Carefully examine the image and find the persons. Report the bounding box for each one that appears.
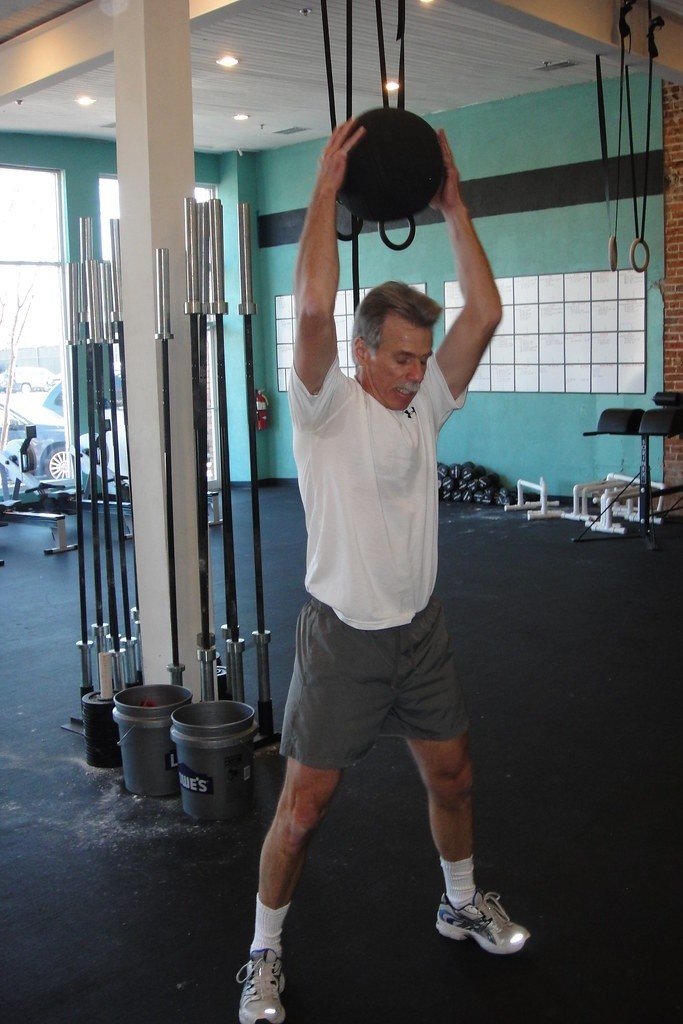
[233,118,533,1024]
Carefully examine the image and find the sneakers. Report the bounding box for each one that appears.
[435,887,532,956]
[235,947,286,1024]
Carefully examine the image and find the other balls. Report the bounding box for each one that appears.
[338,107,444,221]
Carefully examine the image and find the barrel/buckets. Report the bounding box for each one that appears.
[170,700,260,822]
[111,683,193,797]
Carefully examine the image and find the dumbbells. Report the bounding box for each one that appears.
[437,461,539,506]
